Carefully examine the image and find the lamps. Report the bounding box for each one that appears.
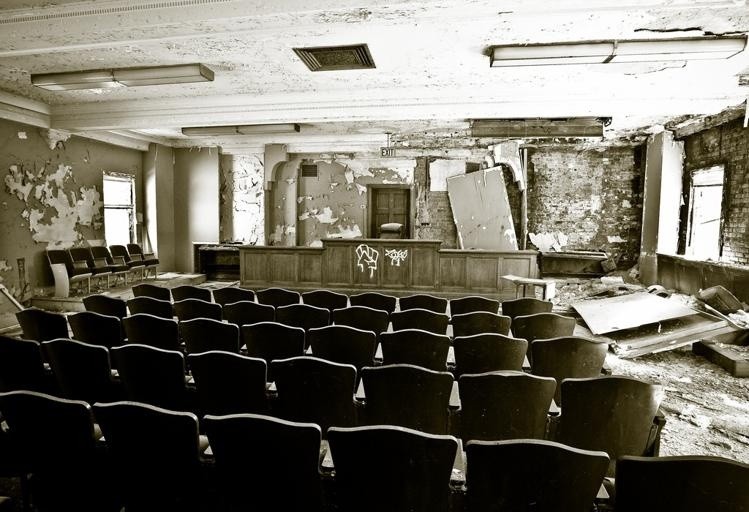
[31,63,214,91]
[490,35,748,67]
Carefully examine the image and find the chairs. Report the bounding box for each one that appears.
[2,387,748,510]
[47,243,162,298]
[3,282,667,460]
